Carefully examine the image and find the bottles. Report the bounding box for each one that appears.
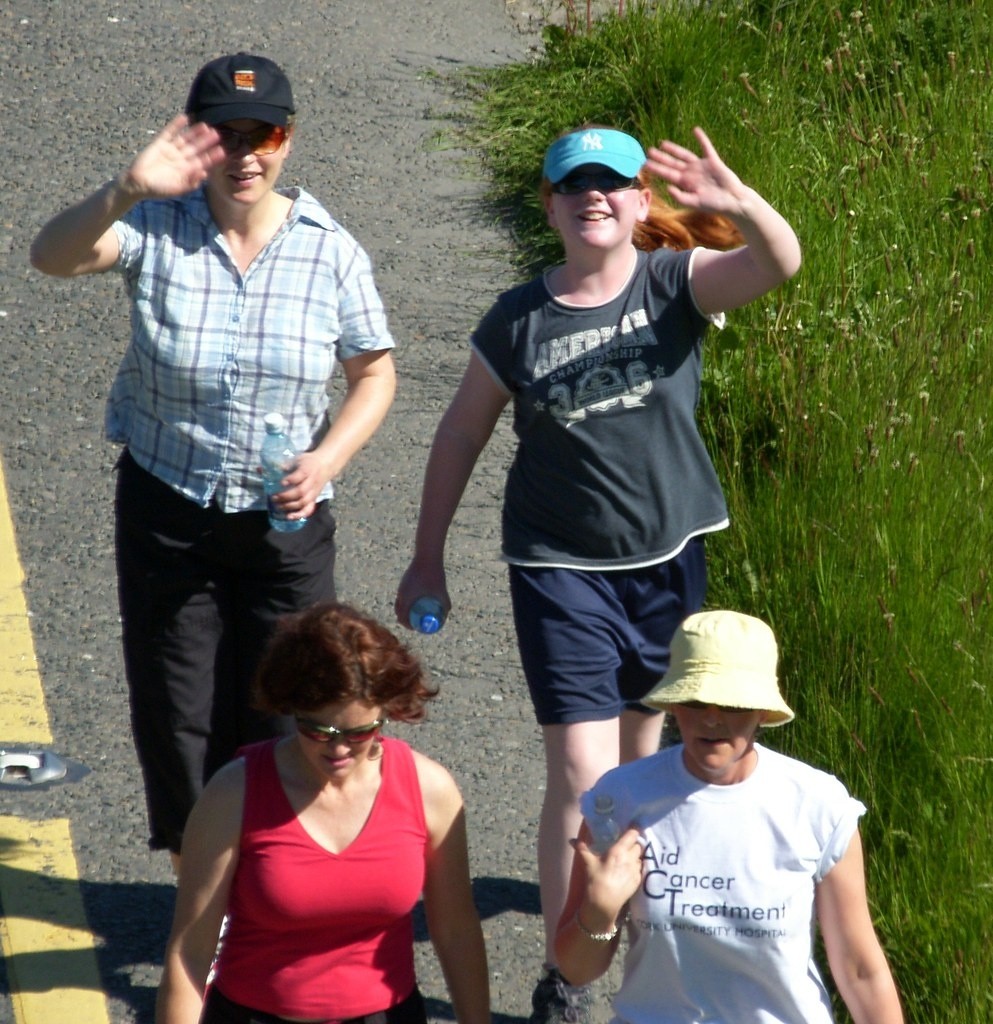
[409,597,445,634]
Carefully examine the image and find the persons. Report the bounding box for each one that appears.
[394,125,801,1024]
[32,52,397,985]
[153,604,491,1024]
[554,610,904,1024]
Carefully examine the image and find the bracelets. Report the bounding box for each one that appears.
[576,909,617,941]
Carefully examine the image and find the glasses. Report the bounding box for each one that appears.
[552,170,639,194]
[295,703,384,743]
[219,125,286,156]
[677,701,754,714]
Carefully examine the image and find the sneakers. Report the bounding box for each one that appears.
[529,963,592,1024]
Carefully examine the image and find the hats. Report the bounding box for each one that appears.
[542,129,646,183]
[639,610,795,727]
[185,50,296,127]
